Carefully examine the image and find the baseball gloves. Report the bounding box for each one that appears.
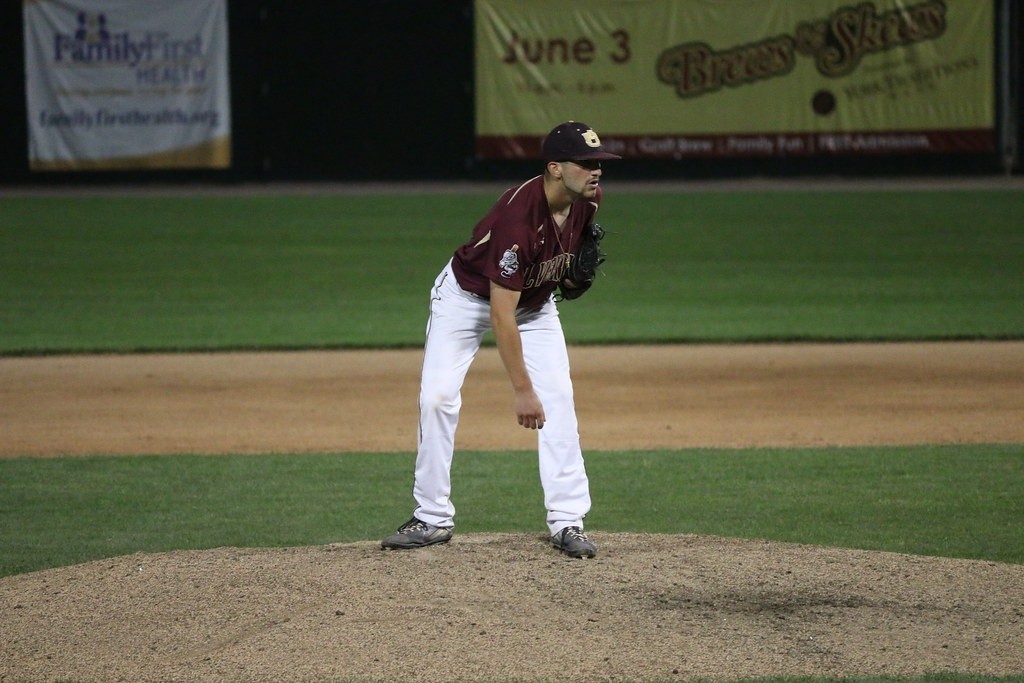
[551,221,608,303]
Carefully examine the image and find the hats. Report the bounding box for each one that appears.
[540,120,623,162]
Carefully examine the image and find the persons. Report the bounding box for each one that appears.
[380,120,621,558]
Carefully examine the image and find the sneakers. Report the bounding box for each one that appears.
[380,516,453,549]
[548,526,598,560]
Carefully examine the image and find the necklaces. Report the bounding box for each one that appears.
[544,178,575,268]
